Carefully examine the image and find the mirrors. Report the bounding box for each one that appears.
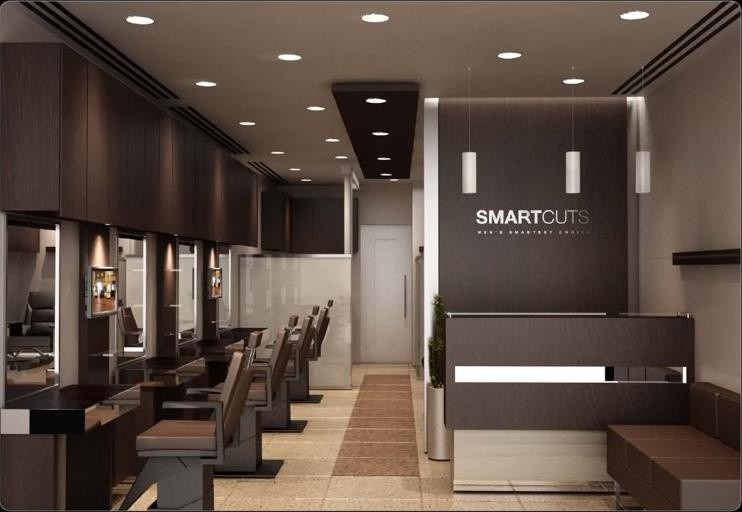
[216,244,232,330]
[112,232,149,356]
[0,214,61,405]
[175,238,198,345]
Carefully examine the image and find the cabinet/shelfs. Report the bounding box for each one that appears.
[0,41,345,254]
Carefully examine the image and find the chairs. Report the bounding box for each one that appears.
[135,300,334,511]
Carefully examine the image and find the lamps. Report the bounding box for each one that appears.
[461,67,477,194]
[565,66,581,194]
[636,64,652,194]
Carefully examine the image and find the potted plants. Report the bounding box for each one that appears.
[427,295,455,462]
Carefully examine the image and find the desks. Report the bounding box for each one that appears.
[0,382,142,512]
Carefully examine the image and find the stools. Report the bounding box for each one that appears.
[606,422,742,512]
